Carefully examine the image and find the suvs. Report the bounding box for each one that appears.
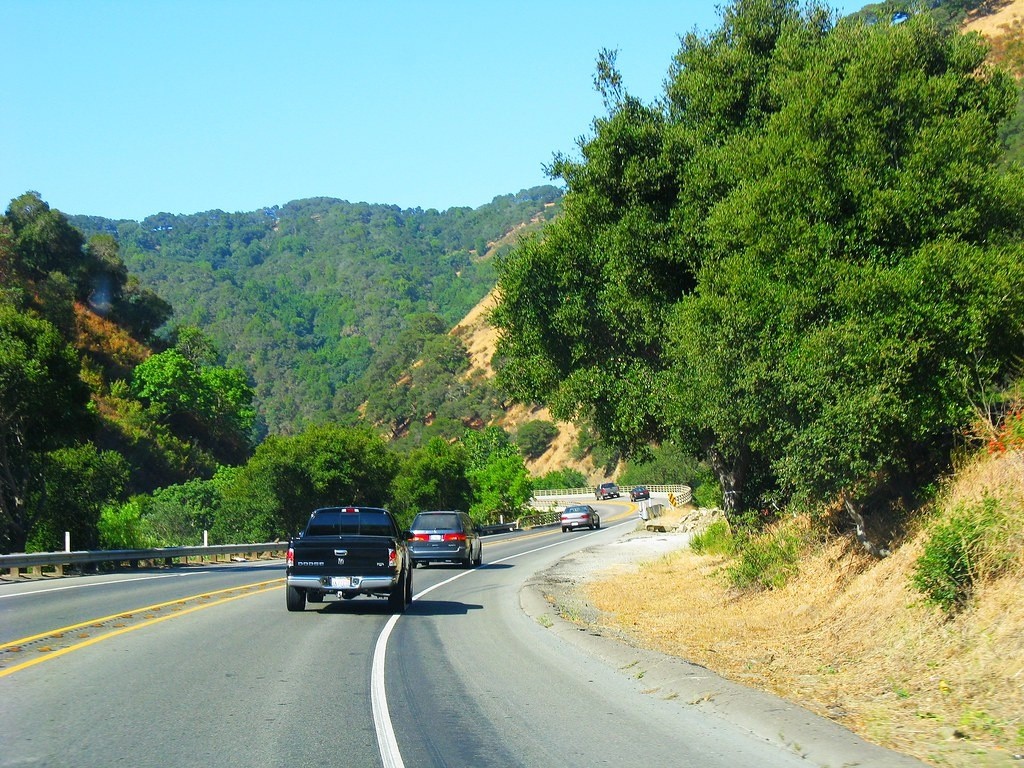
[407,510,482,568]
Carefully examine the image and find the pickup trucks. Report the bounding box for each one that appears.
[286,507,413,612]
[595,482,620,500]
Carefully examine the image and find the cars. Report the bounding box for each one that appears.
[630,487,650,502]
[560,505,601,532]
